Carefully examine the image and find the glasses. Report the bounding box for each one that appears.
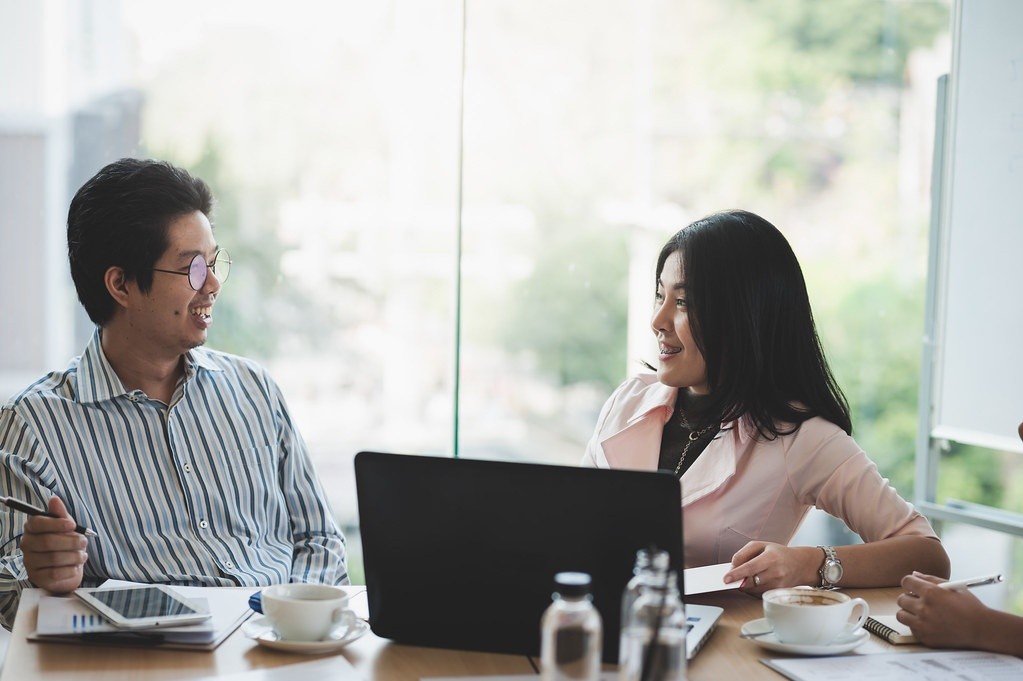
[145,248,232,291]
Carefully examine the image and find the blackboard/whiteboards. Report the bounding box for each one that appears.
[926,0,1023,457]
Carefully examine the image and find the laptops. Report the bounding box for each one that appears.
[353,450,723,661]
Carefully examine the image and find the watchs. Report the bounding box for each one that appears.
[817,545,844,590]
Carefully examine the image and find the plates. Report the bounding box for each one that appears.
[244,616,369,655]
[743,618,869,656]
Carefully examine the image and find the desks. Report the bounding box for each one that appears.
[0,584,1023,681]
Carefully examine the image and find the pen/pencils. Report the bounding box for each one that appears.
[0,494,99,538]
[936,574,1004,587]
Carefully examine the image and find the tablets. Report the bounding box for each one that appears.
[73,583,213,629]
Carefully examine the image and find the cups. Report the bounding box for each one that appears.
[260,583,357,643]
[761,587,869,644]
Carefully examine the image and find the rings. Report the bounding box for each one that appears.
[754,575,760,586]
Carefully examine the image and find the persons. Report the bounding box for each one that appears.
[896,571,1023,657]
[581,212,953,597]
[0,159,350,634]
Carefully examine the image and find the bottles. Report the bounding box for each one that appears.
[540,572,603,681]
[618,549,686,681]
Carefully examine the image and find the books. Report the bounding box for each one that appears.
[857,615,922,645]
[24,579,265,652]
